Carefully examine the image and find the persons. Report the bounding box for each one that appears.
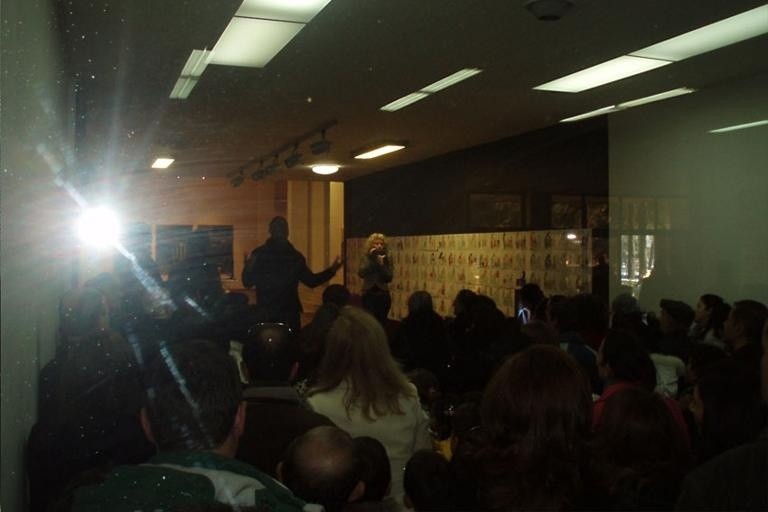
[26,215,768,512]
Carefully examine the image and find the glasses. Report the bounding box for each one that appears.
[227,119,339,187]
